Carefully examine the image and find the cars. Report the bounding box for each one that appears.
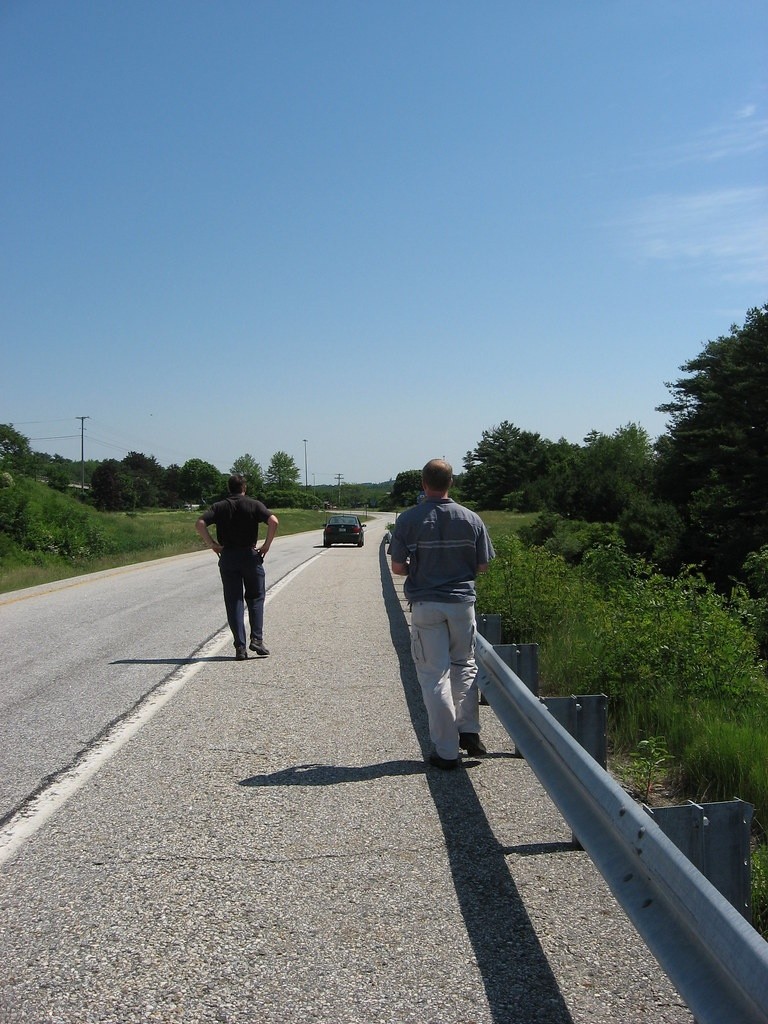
[322,515,367,547]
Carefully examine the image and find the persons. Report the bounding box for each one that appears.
[195,474,279,660]
[386,459,496,768]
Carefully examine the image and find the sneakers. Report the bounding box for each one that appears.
[249,639,270,655]
[235,647,247,660]
[458,732,487,756]
[429,752,458,770]
